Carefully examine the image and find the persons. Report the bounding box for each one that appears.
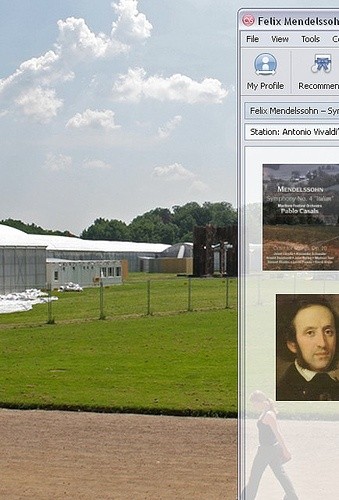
[277,300,339,401]
[237,391,299,500]
